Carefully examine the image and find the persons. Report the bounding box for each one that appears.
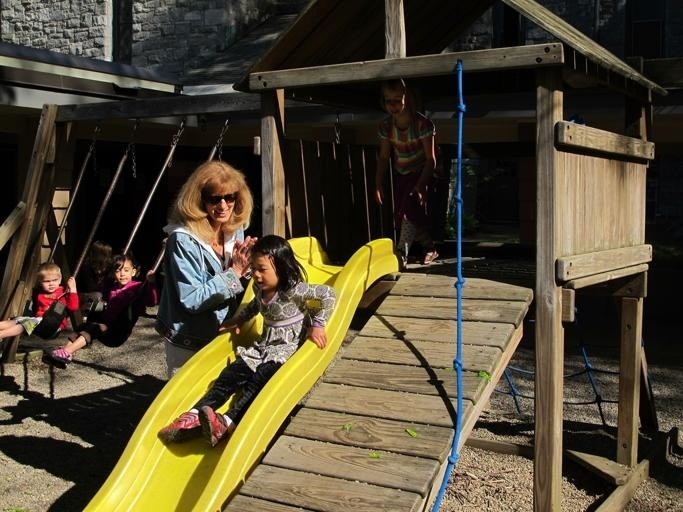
[372,79,439,266]
[48,254,160,365]
[0,262,80,341]
[158,232,337,454]
[151,155,258,383]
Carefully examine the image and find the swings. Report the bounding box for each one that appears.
[23,118,139,347]
[69,117,234,348]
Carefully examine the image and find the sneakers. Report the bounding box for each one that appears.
[401,256,409,265]
[423,252,439,264]
[43,346,72,369]
[158,405,228,448]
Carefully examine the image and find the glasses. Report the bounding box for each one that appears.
[207,194,235,205]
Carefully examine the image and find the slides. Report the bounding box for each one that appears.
[84,235,400,512]
